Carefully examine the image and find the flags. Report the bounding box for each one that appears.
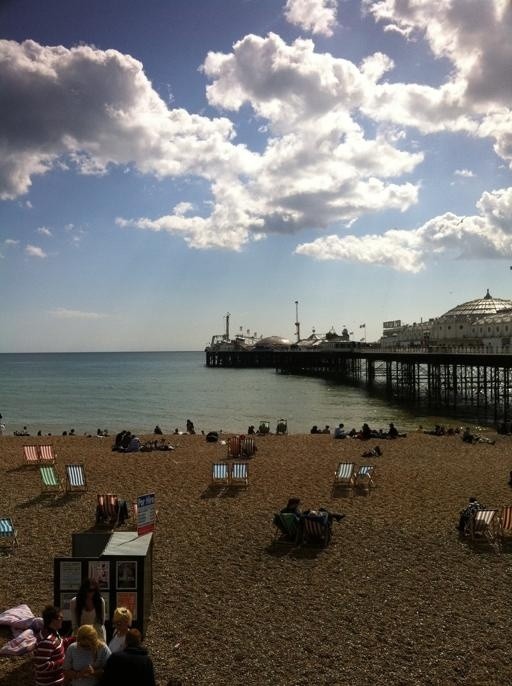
[359,323,365,328]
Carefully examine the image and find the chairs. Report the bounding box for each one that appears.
[272,511,335,549]
[210,436,257,490]
[333,462,355,487]
[96,494,128,531]
[464,510,497,542]
[23,445,88,497]
[493,506,512,541]
[0,518,19,552]
[350,466,377,493]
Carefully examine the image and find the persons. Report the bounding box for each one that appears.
[281,497,301,517]
[455,496,484,531]
[239,418,496,458]
[319,507,346,523]
[14,418,205,453]
[33,577,155,686]
